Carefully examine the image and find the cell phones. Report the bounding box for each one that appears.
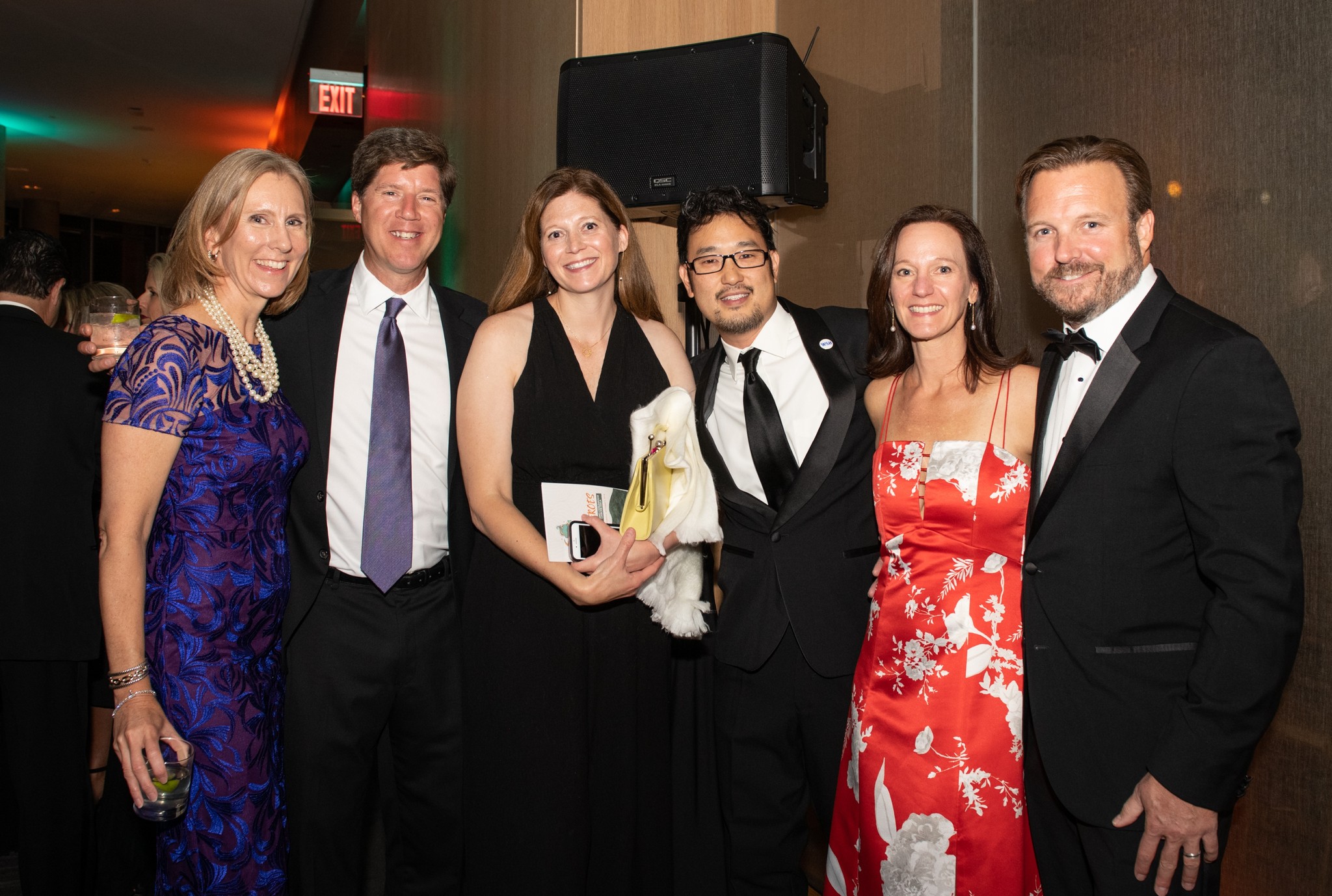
[566,521,622,562]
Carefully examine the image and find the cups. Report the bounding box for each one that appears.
[89,296,140,363]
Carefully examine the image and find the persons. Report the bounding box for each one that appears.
[0,232,114,894]
[59,278,134,338]
[454,166,704,896]
[95,150,316,896]
[675,179,885,896]
[866,136,1300,896]
[76,125,492,896]
[135,251,176,324]
[825,206,1048,896]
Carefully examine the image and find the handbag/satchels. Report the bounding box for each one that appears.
[620,433,672,542]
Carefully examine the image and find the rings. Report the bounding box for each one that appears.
[1183,851,1201,858]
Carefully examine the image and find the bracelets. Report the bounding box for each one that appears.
[106,657,150,689]
[111,689,157,719]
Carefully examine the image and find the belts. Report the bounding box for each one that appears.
[324,559,452,593]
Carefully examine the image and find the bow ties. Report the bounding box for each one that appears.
[1040,327,1101,365]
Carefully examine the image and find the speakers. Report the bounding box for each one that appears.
[556,26,831,220]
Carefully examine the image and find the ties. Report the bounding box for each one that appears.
[360,299,412,596]
[735,348,802,511]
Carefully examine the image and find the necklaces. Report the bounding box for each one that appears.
[194,281,280,404]
[555,293,617,357]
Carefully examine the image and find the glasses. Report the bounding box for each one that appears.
[685,249,770,274]
